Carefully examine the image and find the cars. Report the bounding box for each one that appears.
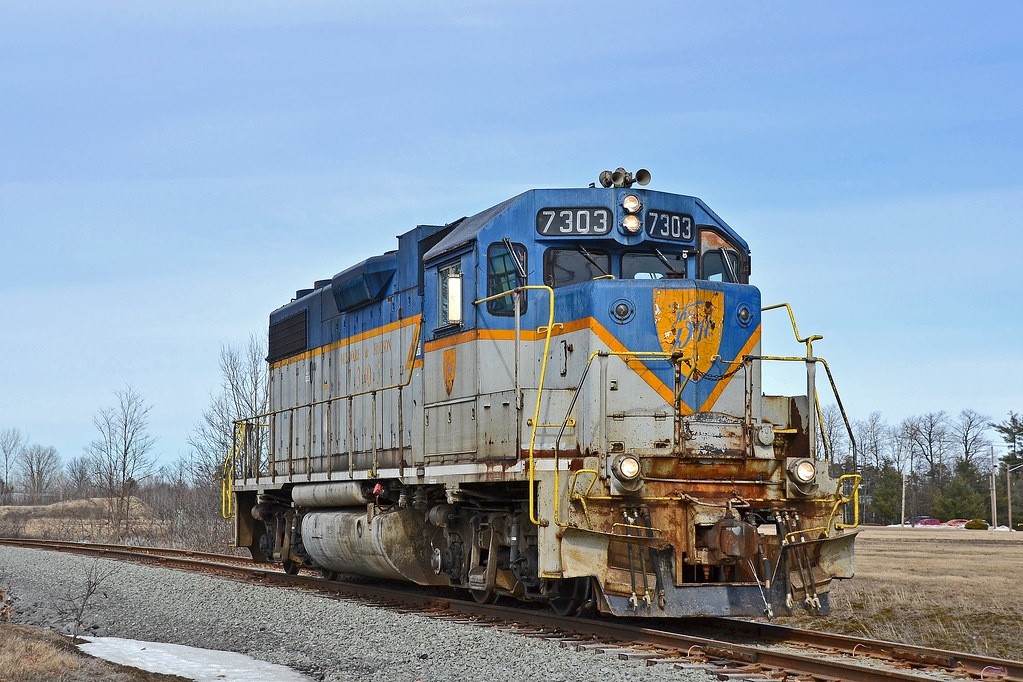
[947,519,968,526]
[917,519,940,526]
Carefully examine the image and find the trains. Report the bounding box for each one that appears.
[228,167,862,630]
